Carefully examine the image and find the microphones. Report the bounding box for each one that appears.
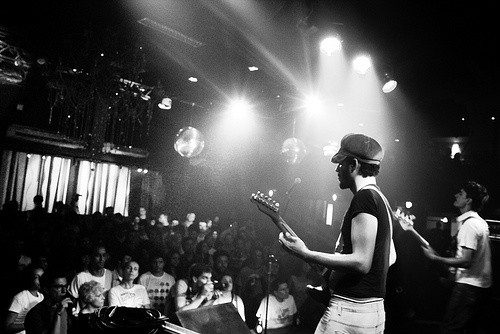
[286,178,301,194]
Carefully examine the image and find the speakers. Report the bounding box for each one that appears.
[171,302,251,334]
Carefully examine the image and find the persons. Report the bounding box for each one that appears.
[68,244,116,304]
[135,255,178,310]
[24,274,76,334]
[167,265,214,322]
[420,183,490,334]
[278,134,393,334]
[107,261,153,317]
[4,266,45,334]
[256,280,297,331]
[211,276,245,322]
[1,195,315,320]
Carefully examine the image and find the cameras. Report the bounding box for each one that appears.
[213,282,229,291]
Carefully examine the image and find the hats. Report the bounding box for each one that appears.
[331,133,384,165]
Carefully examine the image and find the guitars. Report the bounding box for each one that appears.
[392,206,431,248]
[248,190,340,299]
